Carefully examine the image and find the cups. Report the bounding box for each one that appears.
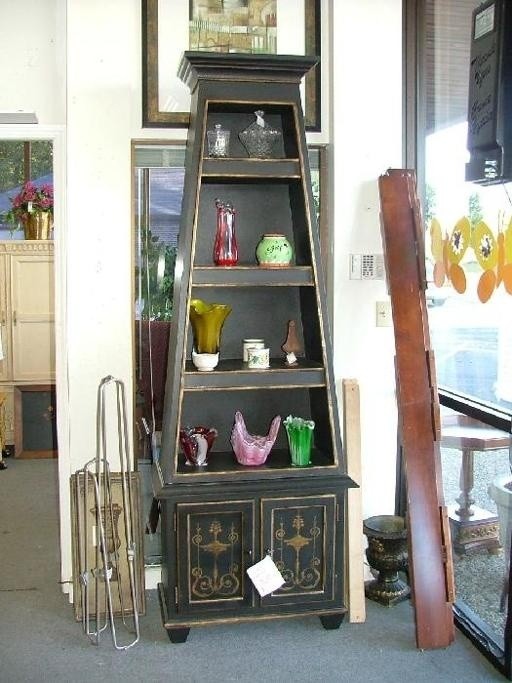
[248,349,270,368]
[281,413,316,468]
[243,338,265,361]
[179,424,218,466]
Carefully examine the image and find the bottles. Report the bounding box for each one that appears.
[214,198,239,265]
[207,123,231,157]
[239,110,282,158]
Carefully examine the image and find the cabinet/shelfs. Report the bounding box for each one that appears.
[0,242,56,387]
[150,49,363,644]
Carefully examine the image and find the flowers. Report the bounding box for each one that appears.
[1,173,54,236]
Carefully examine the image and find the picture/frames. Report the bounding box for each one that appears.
[141,0,326,133]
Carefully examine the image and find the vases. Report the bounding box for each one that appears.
[23,210,48,240]
